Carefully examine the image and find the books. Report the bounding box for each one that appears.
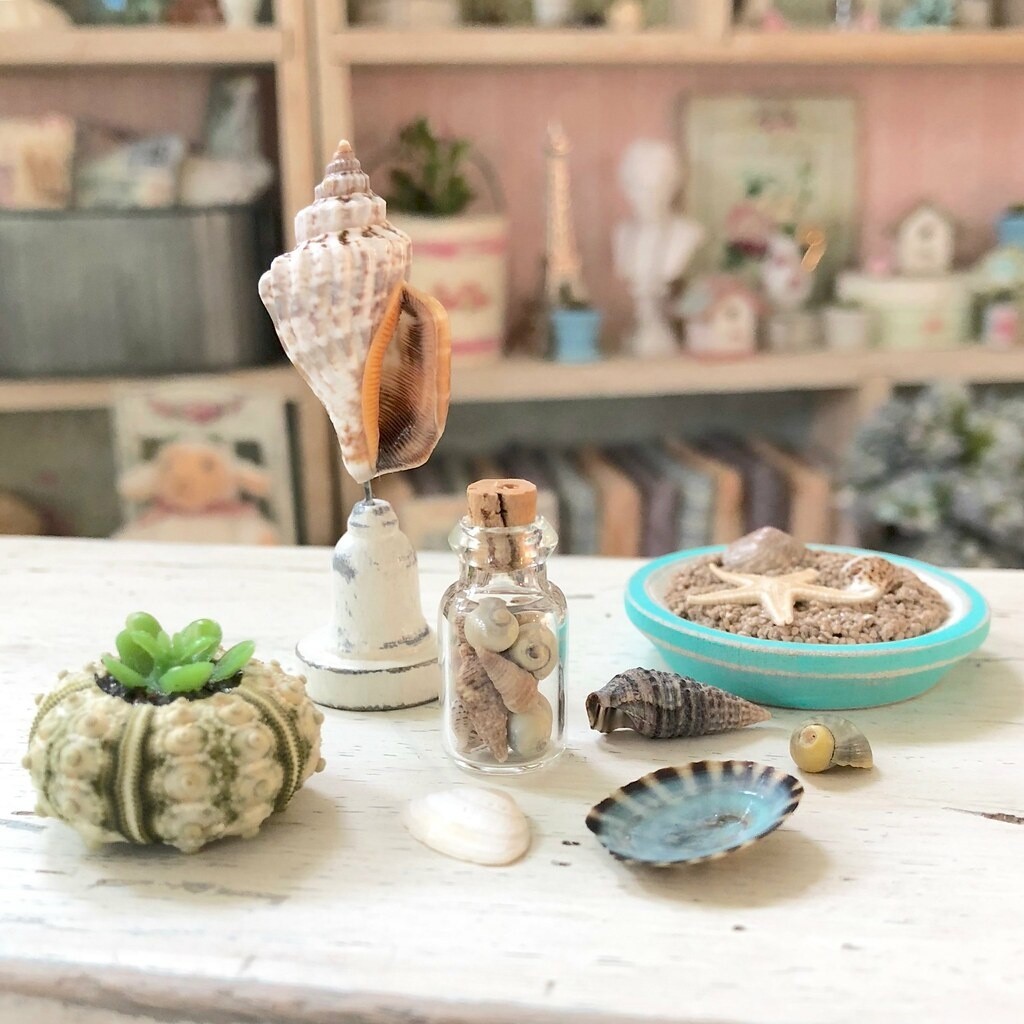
[406,429,850,557]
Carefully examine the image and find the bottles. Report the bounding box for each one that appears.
[439,478,569,770]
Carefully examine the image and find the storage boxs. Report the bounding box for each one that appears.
[0,195,257,386]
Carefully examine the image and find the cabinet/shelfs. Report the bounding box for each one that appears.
[0,1,334,548]
[312,2,1023,570]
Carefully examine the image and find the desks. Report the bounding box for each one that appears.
[1,536,1024,1021]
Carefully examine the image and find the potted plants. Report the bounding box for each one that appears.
[381,114,508,369]
[23,612,326,854]
[816,299,872,348]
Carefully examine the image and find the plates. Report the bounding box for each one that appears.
[625,542,990,712]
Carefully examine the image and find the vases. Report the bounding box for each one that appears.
[553,284,604,365]
